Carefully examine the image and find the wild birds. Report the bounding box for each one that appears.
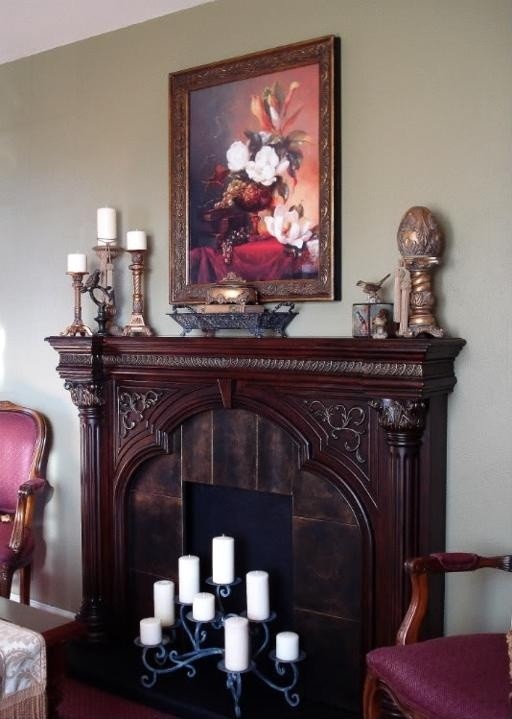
[355,273,391,303]
[355,311,367,333]
[79,269,101,293]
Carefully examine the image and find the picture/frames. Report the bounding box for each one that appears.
[167,34,341,312]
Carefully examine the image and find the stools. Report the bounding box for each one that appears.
[0,616,47,719]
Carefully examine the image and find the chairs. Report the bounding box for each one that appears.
[1,398,59,607]
[359,546,512,718]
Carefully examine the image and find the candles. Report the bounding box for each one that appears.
[65,250,89,274]
[137,526,306,677]
[92,200,118,248]
[124,225,151,252]
